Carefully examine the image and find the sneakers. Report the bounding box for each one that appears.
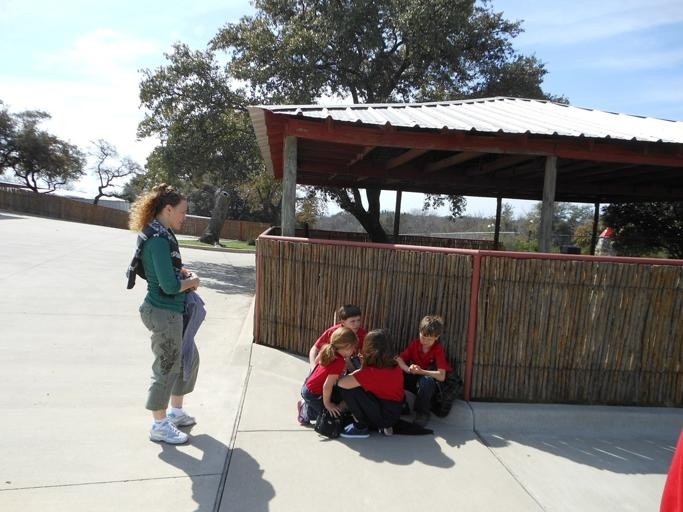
[339,423,370,438]
[414,414,428,426]
[298,400,310,424]
[149,422,188,444]
[168,412,195,426]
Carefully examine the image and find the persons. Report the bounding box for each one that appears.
[308,303,367,375]
[297,326,359,425]
[337,328,404,439]
[396,315,453,427]
[125,181,201,445]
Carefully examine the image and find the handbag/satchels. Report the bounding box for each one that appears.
[431,373,463,417]
[315,409,343,438]
